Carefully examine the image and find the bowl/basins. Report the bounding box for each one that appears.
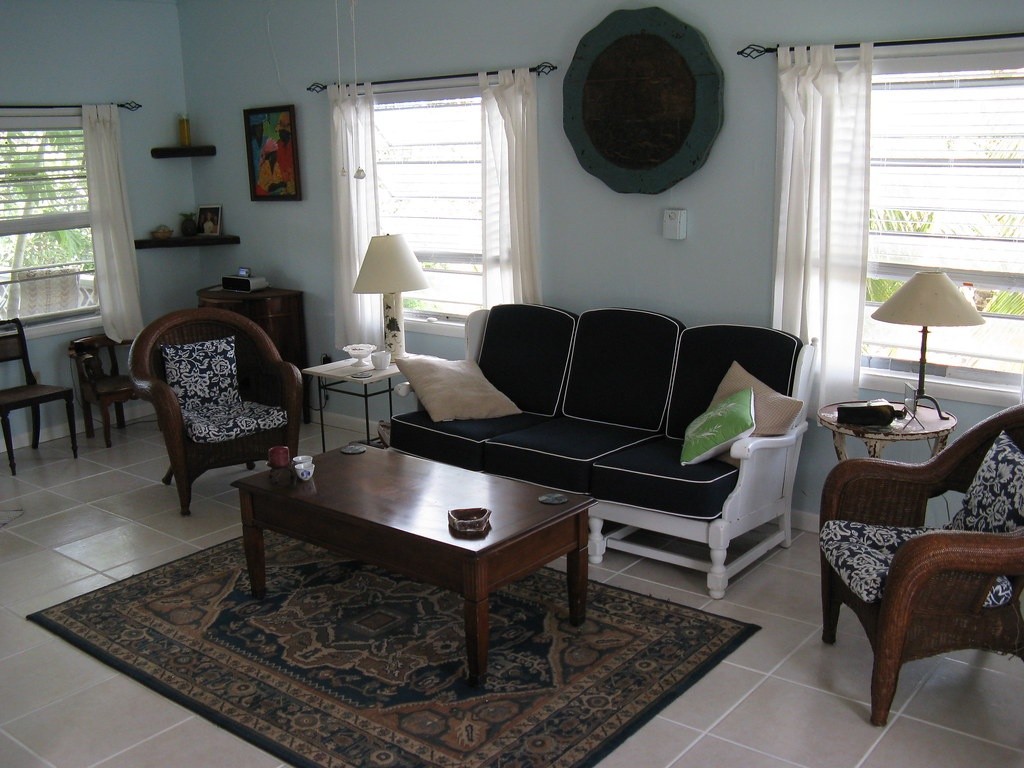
[150,231,173,239]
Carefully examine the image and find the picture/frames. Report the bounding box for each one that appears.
[244,104,302,202]
[196,205,221,235]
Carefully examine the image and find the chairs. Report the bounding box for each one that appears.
[128,306,303,516]
[819,403,1024,726]
[68,333,163,448]
[0,318,78,476]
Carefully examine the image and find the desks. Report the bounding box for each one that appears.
[817,400,957,464]
[302,351,447,454]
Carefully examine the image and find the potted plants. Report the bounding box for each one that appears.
[179,212,197,237]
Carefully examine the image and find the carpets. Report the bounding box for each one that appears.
[25,530,766,768]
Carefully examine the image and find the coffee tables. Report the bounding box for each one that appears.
[230,439,600,687]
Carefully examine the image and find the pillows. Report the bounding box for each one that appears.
[707,361,804,469]
[160,336,242,410]
[945,428,1024,533]
[679,386,757,466]
[396,353,523,423]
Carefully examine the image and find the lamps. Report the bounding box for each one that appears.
[352,234,429,362]
[872,270,981,421]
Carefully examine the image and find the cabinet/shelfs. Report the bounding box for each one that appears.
[196,284,311,425]
[133,145,240,249]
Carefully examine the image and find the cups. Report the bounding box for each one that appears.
[372,352,391,369]
[269,446,288,466]
[293,455,315,480]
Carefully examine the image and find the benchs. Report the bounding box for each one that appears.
[389,305,817,600]
